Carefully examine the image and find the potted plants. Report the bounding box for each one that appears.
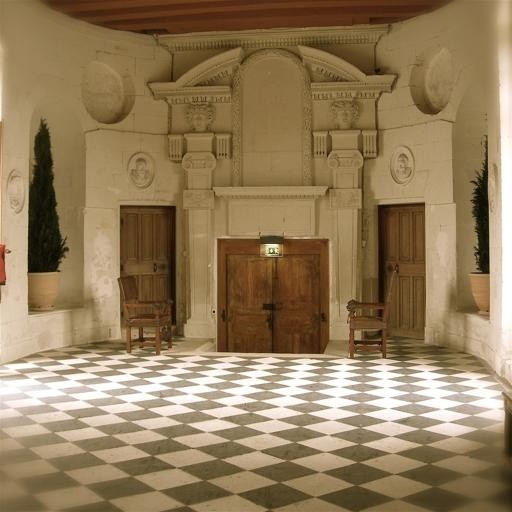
[468,134,490,315]
[27,118,69,311]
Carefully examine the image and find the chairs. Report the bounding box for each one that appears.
[118,276,173,354]
[347,265,400,359]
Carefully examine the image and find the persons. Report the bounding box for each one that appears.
[329,101,360,129]
[187,104,216,132]
